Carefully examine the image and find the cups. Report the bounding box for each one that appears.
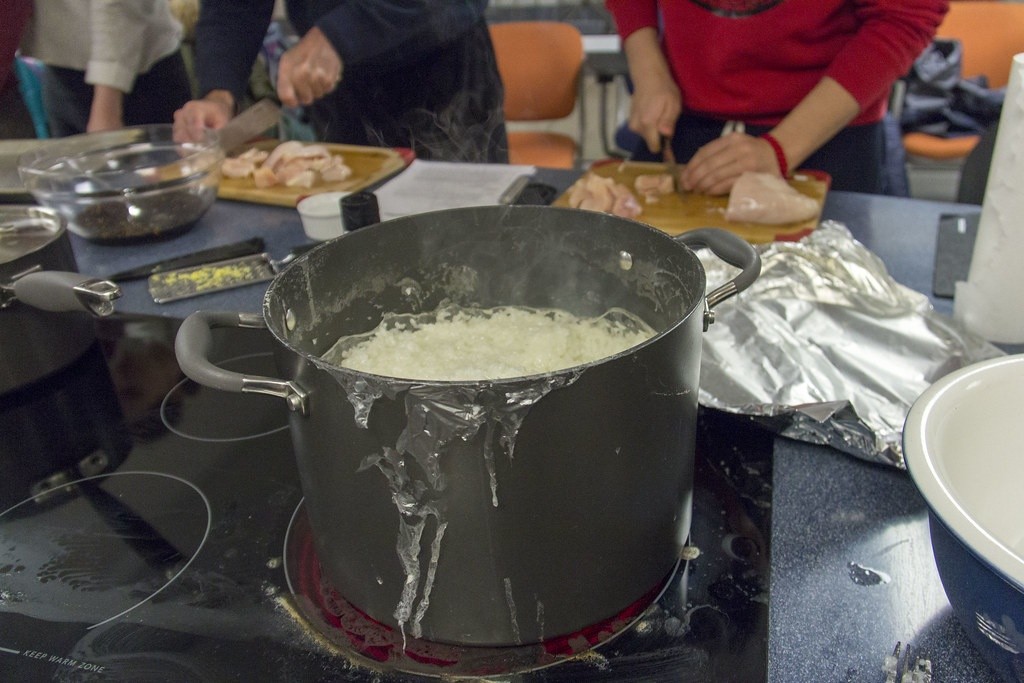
[295,192,349,241]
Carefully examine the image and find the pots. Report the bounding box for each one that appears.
[1,202,120,391]
[170,200,760,648]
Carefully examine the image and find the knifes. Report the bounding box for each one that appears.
[663,133,687,193]
[198,97,285,152]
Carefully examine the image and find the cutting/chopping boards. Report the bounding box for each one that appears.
[540,160,831,249]
[170,134,414,210]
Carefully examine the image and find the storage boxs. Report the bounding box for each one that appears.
[15,119,226,244]
[171,203,764,648]
[902,349,1024,681]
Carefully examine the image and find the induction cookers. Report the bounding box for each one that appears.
[3,310,771,682]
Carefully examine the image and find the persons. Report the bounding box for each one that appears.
[172,0,510,165]
[0,0,191,134]
[599,0,950,198]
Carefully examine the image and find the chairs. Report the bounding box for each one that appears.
[887,75,985,169]
[482,21,588,171]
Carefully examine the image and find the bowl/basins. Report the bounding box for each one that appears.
[16,122,223,245]
[903,353,1023,681]
